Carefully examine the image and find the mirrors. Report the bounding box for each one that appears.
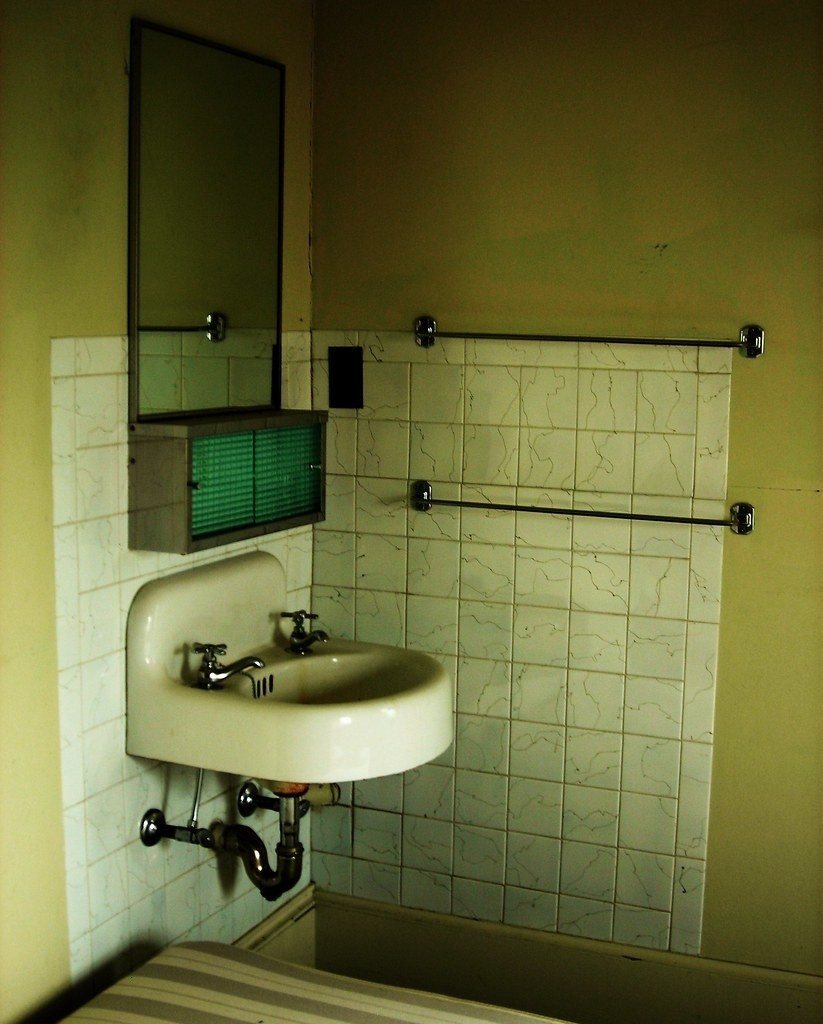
[128,15,288,425]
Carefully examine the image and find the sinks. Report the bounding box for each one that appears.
[235,652,431,709]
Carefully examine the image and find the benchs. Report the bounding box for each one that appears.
[58,938,575,1024]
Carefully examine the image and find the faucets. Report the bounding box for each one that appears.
[279,609,330,652]
[191,641,266,690]
